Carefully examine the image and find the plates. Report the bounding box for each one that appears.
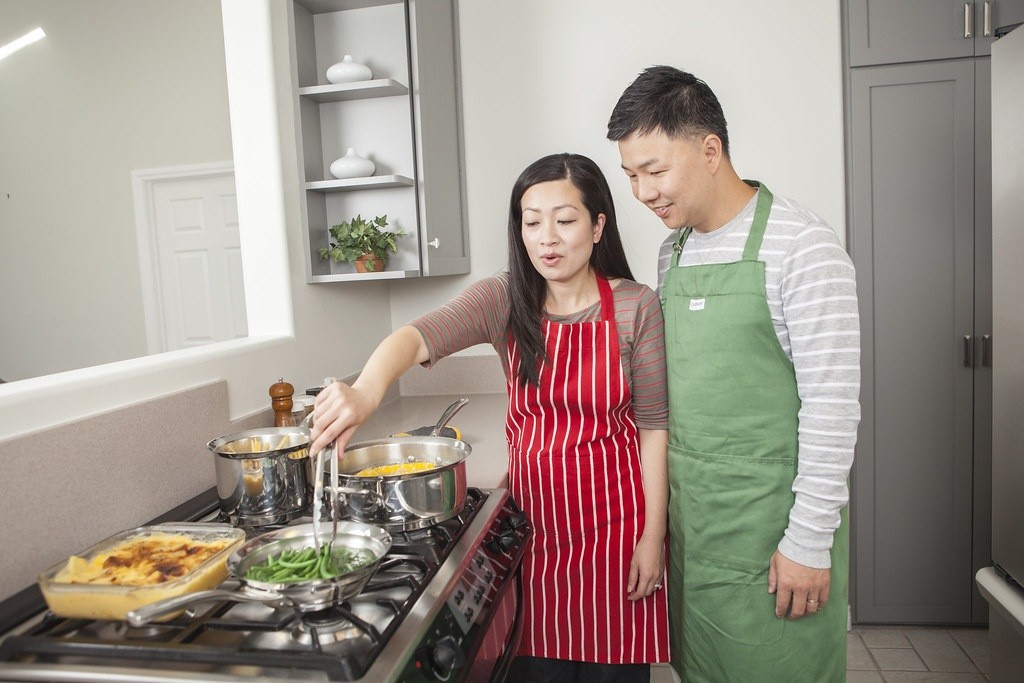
[126,520,394,631]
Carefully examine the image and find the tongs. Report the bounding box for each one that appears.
[313,377,340,547]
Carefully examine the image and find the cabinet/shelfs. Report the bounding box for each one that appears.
[270,0,471,279]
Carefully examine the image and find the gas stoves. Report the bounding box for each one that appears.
[1,430,533,683]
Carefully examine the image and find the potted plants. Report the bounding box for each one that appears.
[322,214,405,274]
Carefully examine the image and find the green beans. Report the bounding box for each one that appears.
[244,540,375,585]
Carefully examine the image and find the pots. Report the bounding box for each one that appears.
[206,407,317,527]
[314,394,471,534]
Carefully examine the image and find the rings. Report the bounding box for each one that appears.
[655,583,662,590]
[807,599,818,603]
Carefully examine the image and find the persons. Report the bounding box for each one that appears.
[607,66,861,683]
[309,153,671,683]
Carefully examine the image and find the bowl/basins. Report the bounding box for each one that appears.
[34,523,246,623]
